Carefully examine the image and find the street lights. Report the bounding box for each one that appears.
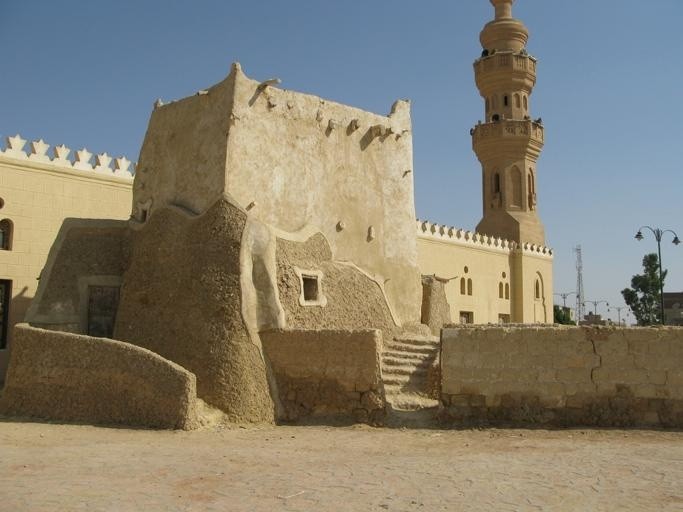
[634,225,679,324]
